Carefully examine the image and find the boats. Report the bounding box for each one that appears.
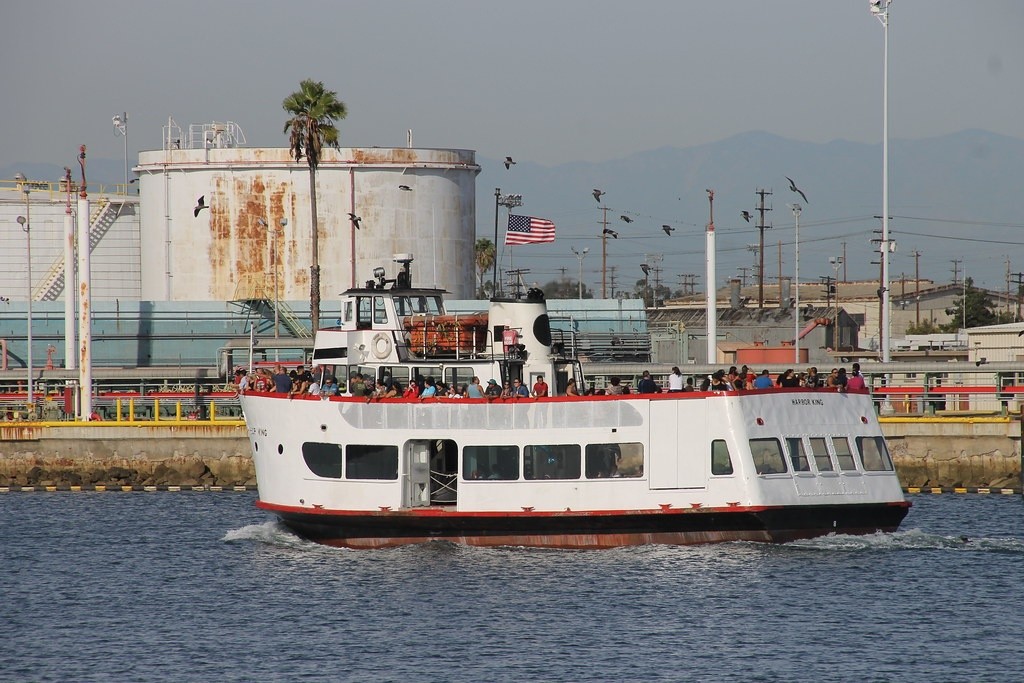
[235,249,912,551]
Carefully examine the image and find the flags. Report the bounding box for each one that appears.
[505,214,556,245]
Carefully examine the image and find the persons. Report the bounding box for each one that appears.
[531,376,548,400]
[402,377,468,401]
[500,381,513,399]
[581,388,595,396]
[349,372,403,403]
[700,365,774,391]
[597,377,630,395]
[487,464,643,479]
[485,379,502,399]
[667,367,686,392]
[827,368,848,387]
[512,378,529,397]
[685,377,694,392]
[235,365,340,396]
[637,371,658,394]
[847,363,865,389]
[566,378,579,396]
[466,377,485,398]
[776,367,819,388]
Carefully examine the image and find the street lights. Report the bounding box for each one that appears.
[574,247,590,299]
[792,203,800,364]
[14,171,33,420]
[112,111,129,195]
[871,0,894,416]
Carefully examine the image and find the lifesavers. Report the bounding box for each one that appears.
[28,413,37,421]
[371,332,392,359]
